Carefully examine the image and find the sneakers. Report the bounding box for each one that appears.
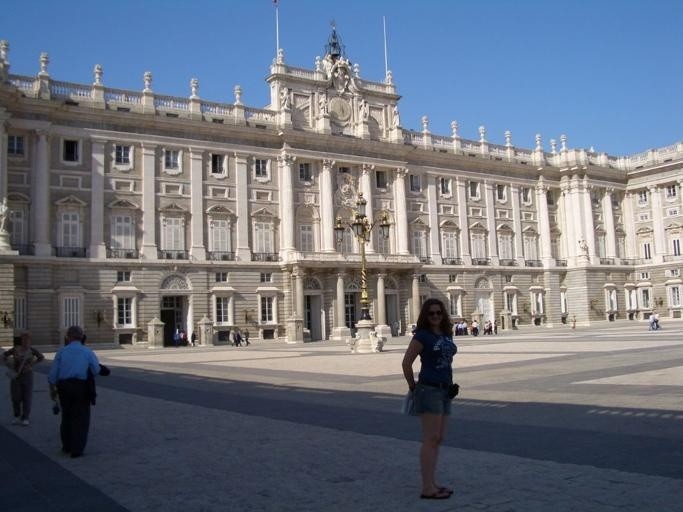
[11,417,30,426]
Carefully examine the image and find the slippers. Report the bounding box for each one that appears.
[420,487,454,500]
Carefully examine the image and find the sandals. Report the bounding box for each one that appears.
[417,380,449,390]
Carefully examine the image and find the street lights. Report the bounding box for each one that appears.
[334,192,389,320]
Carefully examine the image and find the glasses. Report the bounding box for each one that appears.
[427,310,442,316]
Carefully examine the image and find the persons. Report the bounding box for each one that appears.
[191,332,197,347]
[47,327,101,458]
[649,311,662,331]
[0,311,15,329]
[401,299,457,499]
[388,318,399,337]
[173,324,188,347]
[1,331,45,426]
[571,315,577,329]
[452,318,498,337]
[231,328,251,347]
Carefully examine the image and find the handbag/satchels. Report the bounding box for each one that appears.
[4,368,18,379]
[448,383,459,399]
